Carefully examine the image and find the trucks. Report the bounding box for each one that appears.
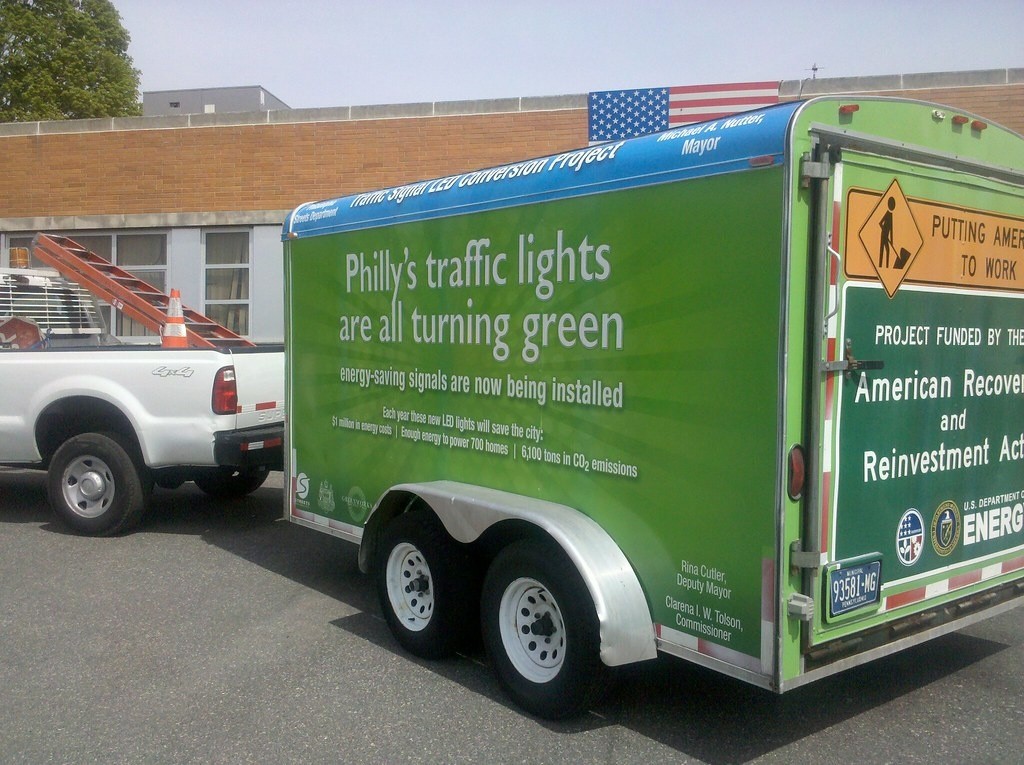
[276,93,1023,720]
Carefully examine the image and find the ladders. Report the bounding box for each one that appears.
[30,231,257,352]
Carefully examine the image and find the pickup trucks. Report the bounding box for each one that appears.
[0,246,285,539]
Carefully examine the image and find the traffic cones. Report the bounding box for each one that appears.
[160,287,188,350]
